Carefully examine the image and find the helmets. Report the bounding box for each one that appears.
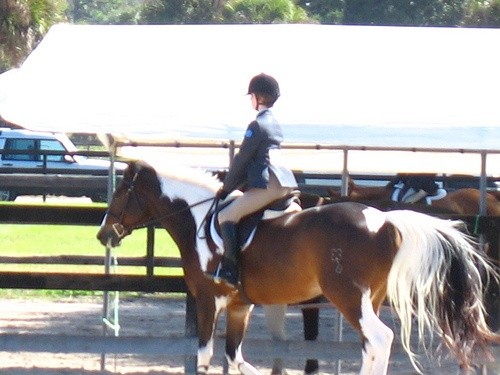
[246,73,281,97]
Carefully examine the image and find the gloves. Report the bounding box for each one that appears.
[215,188,227,200]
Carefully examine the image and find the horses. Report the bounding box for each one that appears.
[95,156,500,375]
[327,175,499,232]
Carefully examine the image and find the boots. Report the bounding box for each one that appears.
[202,221,240,289]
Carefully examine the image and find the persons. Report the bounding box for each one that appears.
[203,73,298,289]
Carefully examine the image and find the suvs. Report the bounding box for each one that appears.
[0,124,128,203]
